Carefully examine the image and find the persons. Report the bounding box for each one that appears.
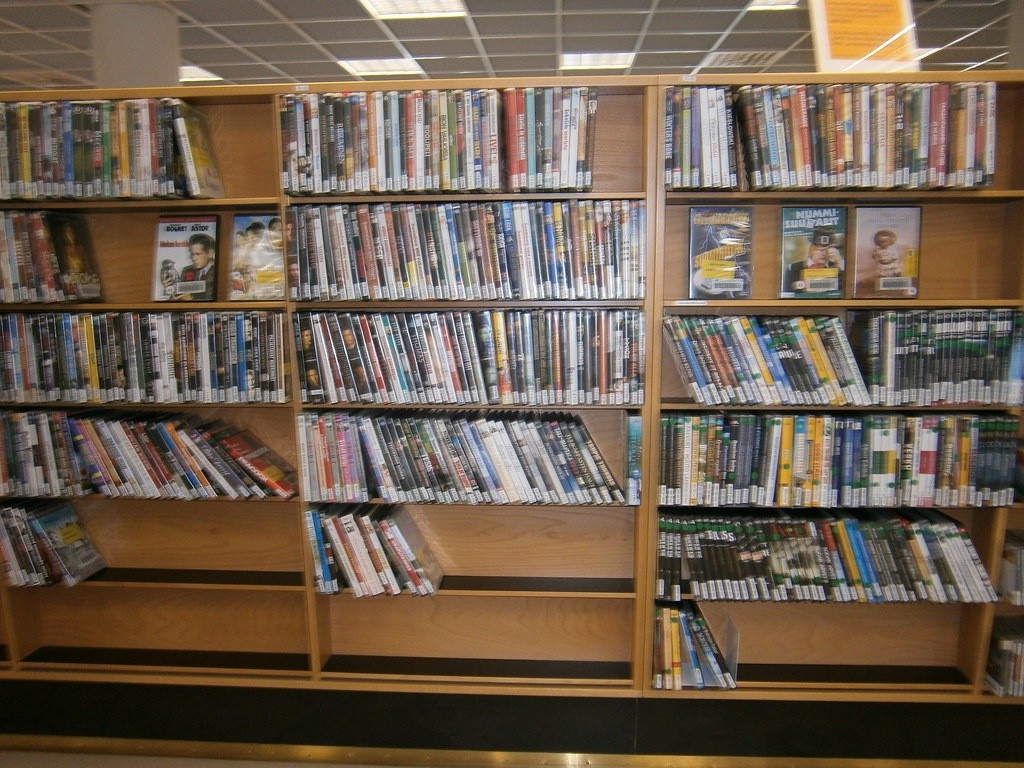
[230,219,367,388]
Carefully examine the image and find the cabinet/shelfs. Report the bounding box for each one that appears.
[0,68,1024,704]
